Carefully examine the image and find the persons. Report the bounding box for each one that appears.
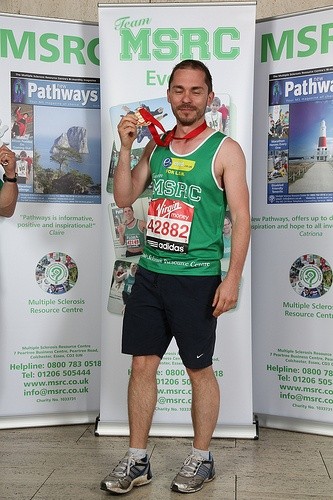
[35,251,78,295]
[268,108,289,181]
[217,104,230,132]
[203,96,224,133]
[119,106,153,144]
[98,58,251,495]
[289,253,333,299]
[221,215,233,259]
[117,205,148,258]
[120,104,164,138]
[14,150,29,184]
[110,262,139,314]
[25,156,33,175]
[107,149,154,190]
[0,145,19,217]
[11,106,32,139]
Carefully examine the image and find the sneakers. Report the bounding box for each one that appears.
[97,453,155,497]
[170,454,218,494]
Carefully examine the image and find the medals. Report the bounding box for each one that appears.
[131,107,150,127]
[119,223,126,230]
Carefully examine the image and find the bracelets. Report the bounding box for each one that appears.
[2,173,18,184]
